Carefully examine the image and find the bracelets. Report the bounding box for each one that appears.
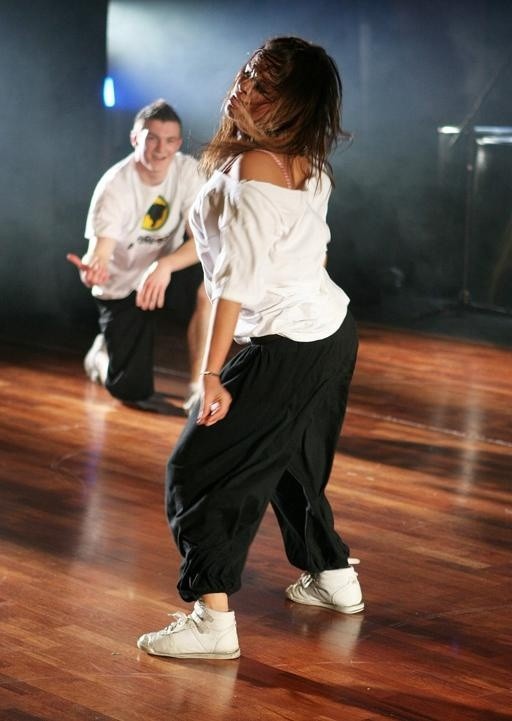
[203,369,221,378]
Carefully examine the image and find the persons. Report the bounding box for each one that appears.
[63,99,217,405]
[137,37,367,663]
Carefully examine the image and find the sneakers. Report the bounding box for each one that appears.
[136,599,241,660]
[285,565,366,614]
